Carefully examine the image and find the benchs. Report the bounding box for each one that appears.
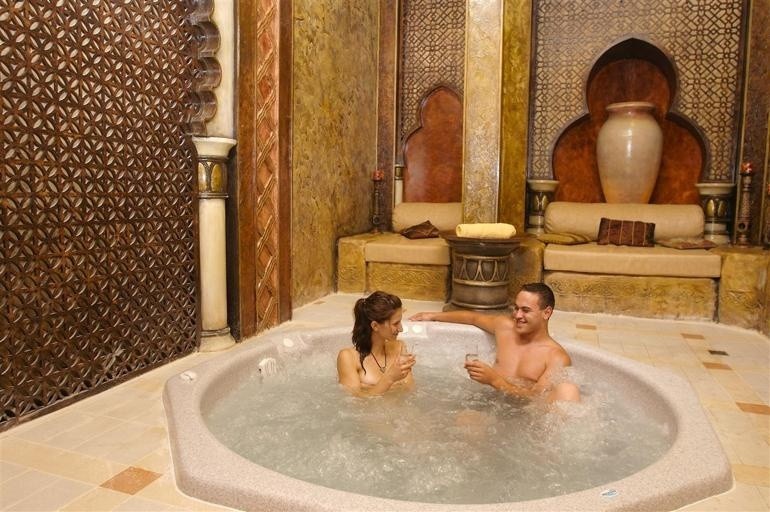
[540,201,723,322]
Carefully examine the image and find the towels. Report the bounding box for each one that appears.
[454,219,518,240]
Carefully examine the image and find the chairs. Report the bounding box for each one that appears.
[336,201,463,302]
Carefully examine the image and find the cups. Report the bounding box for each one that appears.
[398,346,415,373]
[463,346,481,373]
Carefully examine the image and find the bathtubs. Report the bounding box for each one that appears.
[162,320,734,512]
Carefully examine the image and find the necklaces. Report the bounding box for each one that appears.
[369,342,388,375]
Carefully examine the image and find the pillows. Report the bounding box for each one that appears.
[654,237,719,250]
[597,216,656,248]
[538,232,595,245]
[398,219,440,240]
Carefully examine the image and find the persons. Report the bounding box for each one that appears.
[336,291,416,400]
[407,282,582,428]
